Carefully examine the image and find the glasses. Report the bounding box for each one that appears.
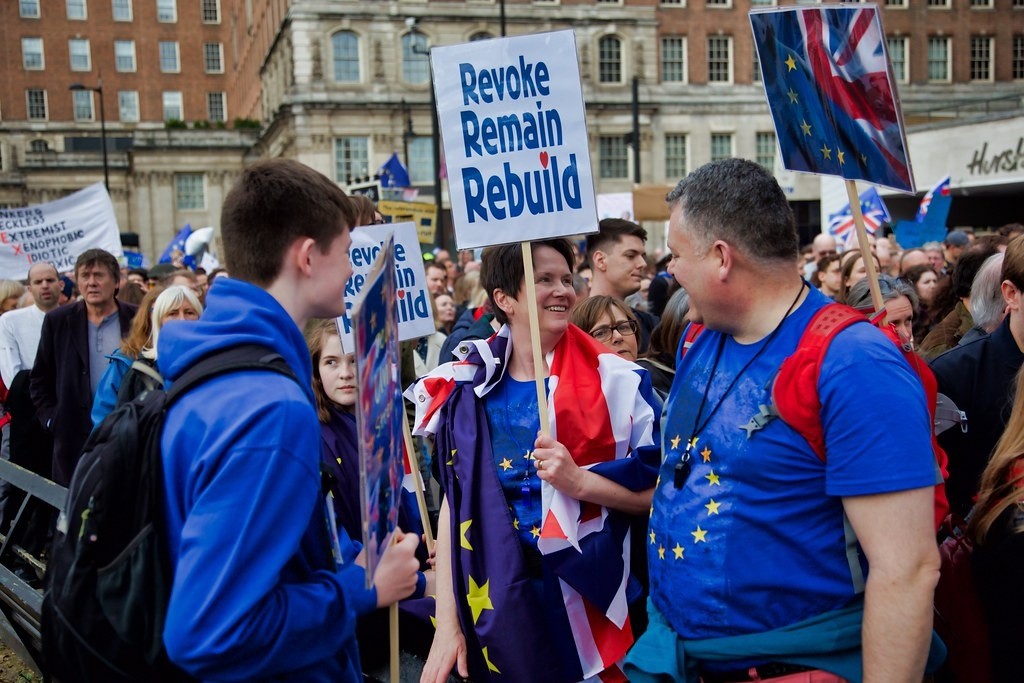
[589,320,637,342]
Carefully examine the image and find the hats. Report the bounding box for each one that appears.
[146,264,174,281]
[945,231,968,246]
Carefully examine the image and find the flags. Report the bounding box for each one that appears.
[828,187,897,249]
[158,224,200,275]
[374,154,409,191]
[918,177,955,231]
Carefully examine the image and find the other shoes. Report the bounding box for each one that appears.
[14,564,40,589]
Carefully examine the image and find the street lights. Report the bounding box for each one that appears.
[71,84,109,191]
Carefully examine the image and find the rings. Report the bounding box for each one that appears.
[538,461,545,468]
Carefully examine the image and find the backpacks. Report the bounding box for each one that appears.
[681,303,951,537]
[933,511,994,683]
[40,343,322,683]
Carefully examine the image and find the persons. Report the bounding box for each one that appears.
[0,157,1024,683]
